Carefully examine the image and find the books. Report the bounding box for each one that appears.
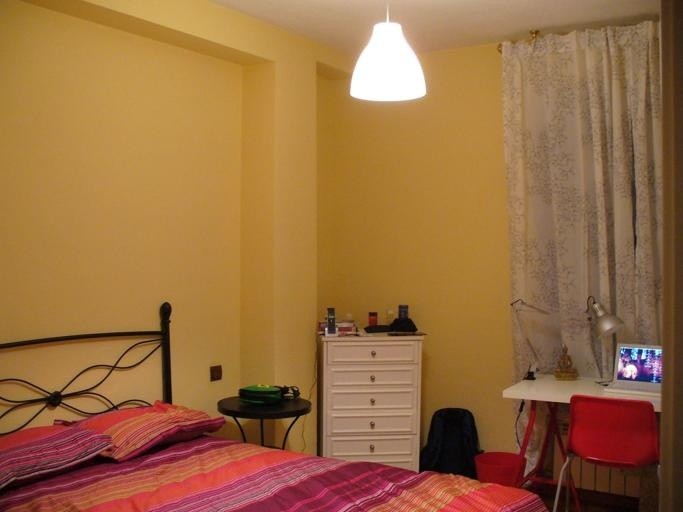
[318,320,361,336]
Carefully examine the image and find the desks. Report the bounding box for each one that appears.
[501,374,659,512]
[217,384,311,450]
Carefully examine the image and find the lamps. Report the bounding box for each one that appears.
[510,293,626,381]
[347,1,426,101]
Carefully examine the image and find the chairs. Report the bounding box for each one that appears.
[550,396,659,511]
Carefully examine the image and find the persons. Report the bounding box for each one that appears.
[617,349,662,380]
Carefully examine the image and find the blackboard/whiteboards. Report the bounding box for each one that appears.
[325,308,338,337]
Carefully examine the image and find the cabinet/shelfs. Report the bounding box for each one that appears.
[319,329,426,473]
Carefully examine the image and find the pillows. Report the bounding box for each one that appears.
[52,402,225,462]
[1,424,112,496]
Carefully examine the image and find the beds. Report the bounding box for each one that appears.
[0,304,546,512]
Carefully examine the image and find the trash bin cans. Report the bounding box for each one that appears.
[473,452,527,488]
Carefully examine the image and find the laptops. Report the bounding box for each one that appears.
[604,343,663,396]
